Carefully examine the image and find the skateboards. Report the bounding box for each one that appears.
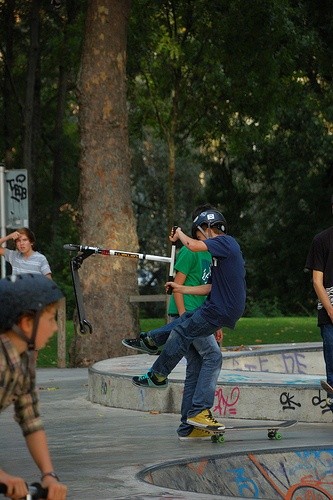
[208,419,299,443]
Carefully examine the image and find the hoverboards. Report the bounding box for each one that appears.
[63,224,178,337]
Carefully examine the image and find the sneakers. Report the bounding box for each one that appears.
[179,428,215,441]
[122,331,158,355]
[132,368,168,390]
[186,407,225,432]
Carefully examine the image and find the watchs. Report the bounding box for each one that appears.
[41,472,60,482]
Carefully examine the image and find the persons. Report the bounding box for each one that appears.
[122,210,247,390]
[0,227,52,281]
[304,225,333,401]
[167,204,225,442]
[0,273,68,500]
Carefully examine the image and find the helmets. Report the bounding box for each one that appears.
[191,209,228,239]
[0,272,63,332]
